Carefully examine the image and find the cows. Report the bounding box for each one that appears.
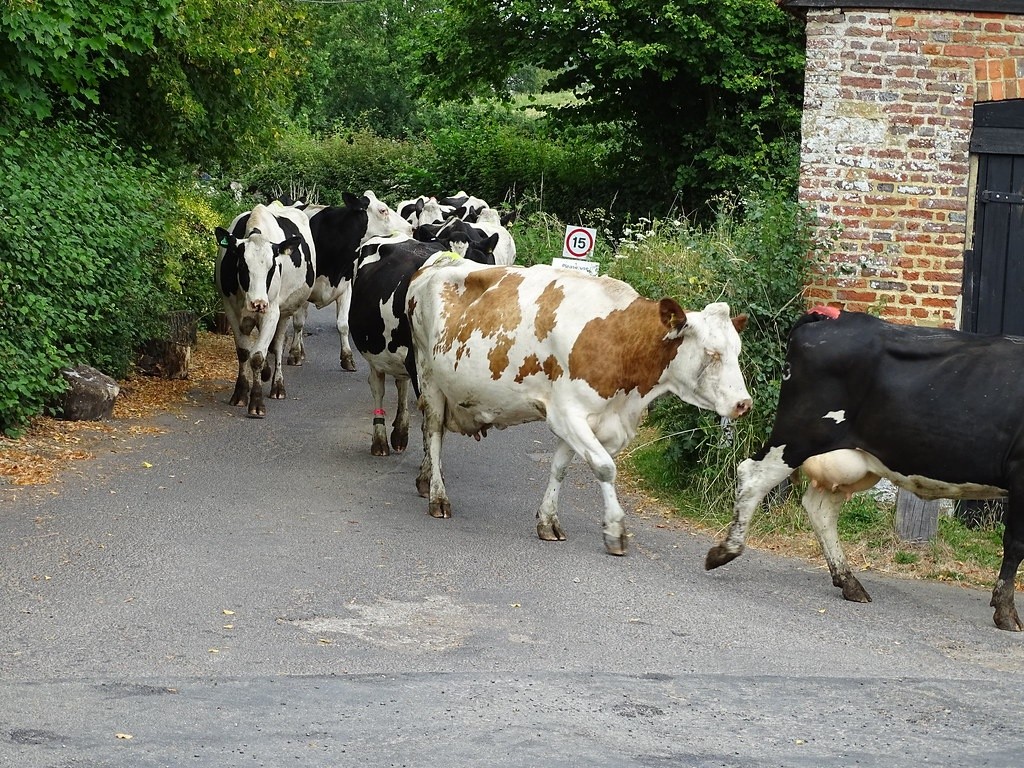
[406,249,753,557]
[350,237,448,456]
[291,188,518,373]
[702,303,1024,632]
[209,199,317,420]
